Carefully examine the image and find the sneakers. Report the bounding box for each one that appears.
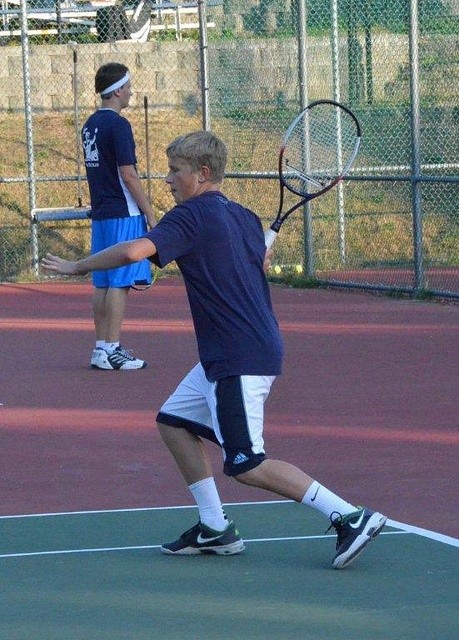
[324,505,387,569]
[91,344,146,370]
[160,517,246,556]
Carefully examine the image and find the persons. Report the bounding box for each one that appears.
[81,63,162,372]
[39,133,385,570]
